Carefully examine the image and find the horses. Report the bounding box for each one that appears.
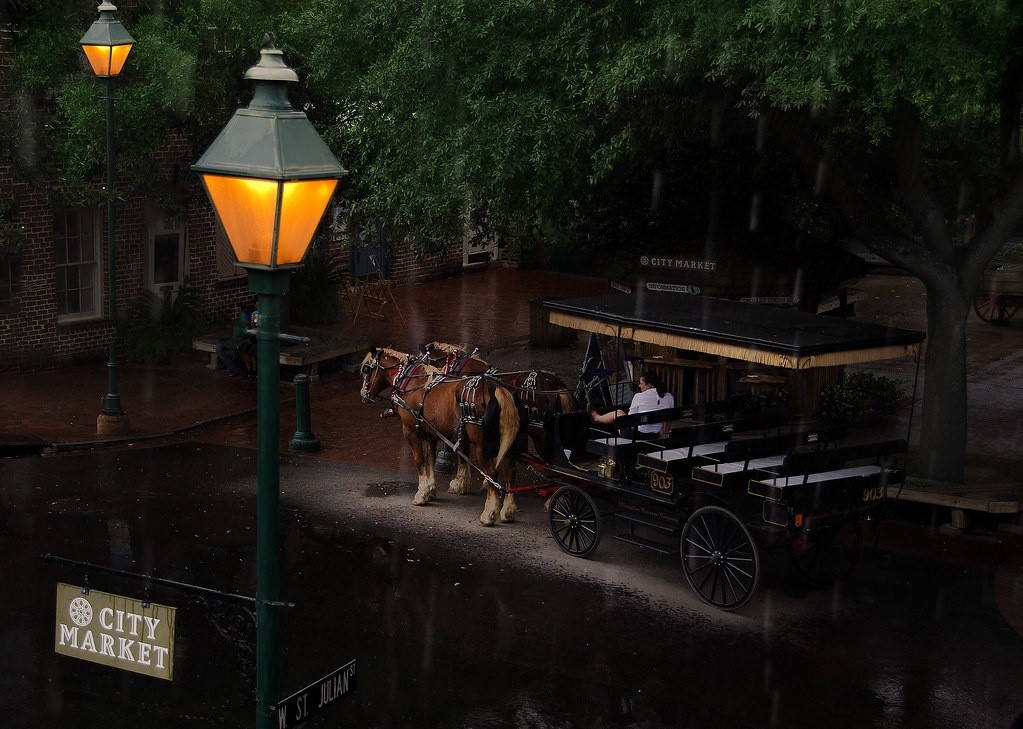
[359,341,576,527]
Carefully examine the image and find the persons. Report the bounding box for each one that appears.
[216,301,260,380]
[586,373,675,440]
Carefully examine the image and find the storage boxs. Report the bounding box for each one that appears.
[979,272,1023,292]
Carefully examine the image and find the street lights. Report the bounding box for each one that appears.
[78,2,136,436]
[187,48,353,729]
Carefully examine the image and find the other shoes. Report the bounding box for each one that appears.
[587,402,597,424]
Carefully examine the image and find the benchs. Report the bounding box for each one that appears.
[748,438,907,529]
[888,475,1023,527]
[191,325,372,381]
[583,398,739,467]
[687,427,834,504]
[633,427,769,495]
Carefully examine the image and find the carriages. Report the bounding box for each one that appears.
[357,284,930,615]
